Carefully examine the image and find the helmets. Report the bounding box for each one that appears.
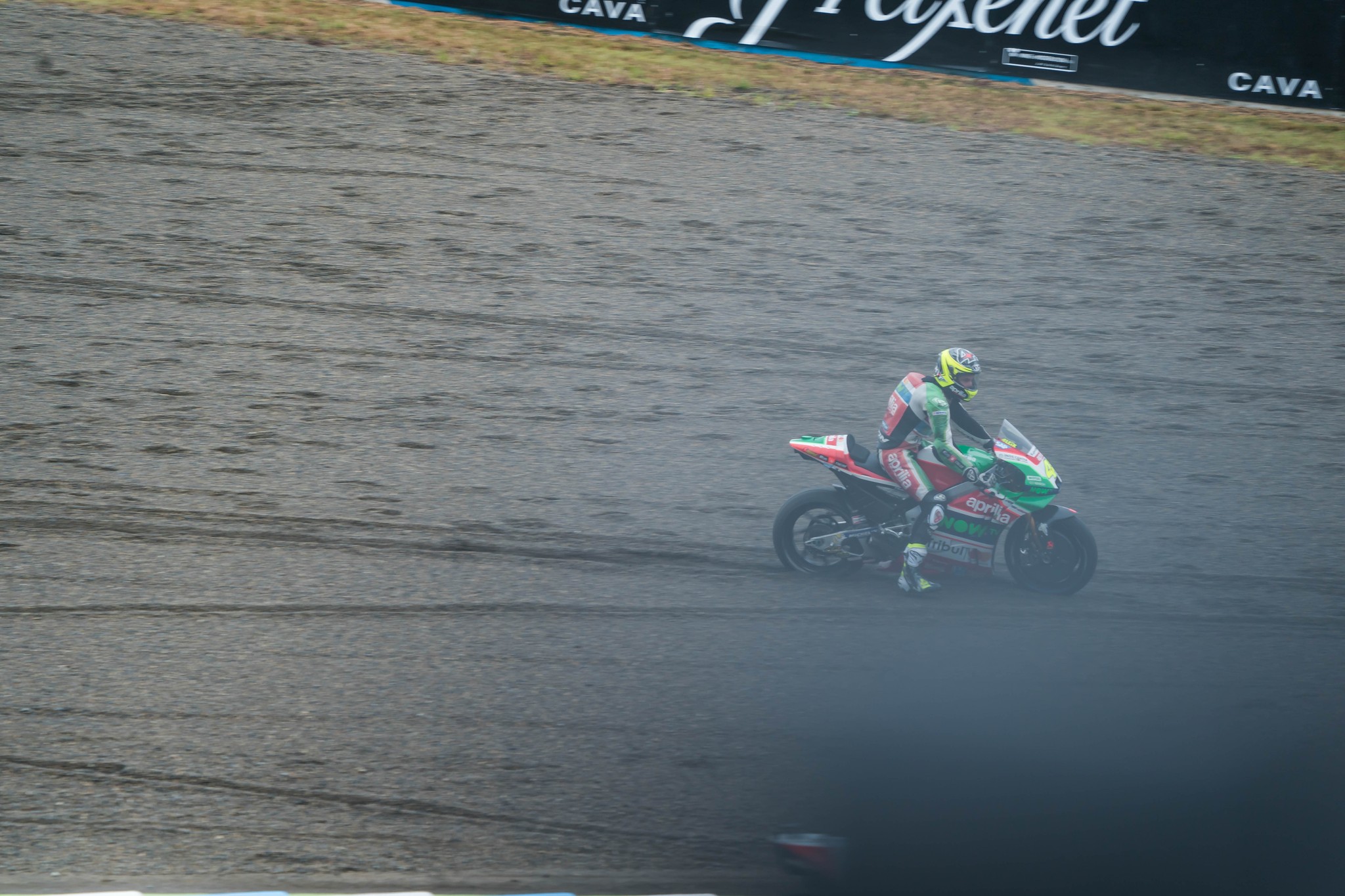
[933,348,981,401]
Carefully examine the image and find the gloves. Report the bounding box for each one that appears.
[979,473,997,489]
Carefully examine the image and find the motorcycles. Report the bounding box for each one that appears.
[773,418,1098,598]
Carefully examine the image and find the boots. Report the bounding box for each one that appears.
[897,543,942,595]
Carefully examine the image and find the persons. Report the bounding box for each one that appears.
[877,347,998,595]
